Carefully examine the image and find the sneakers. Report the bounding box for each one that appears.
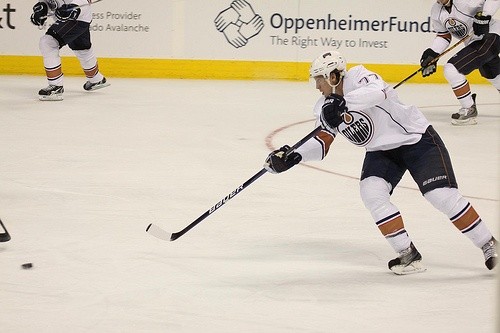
[388,240,426,276]
[450,93,479,126]
[480,235,500,273]
[83,75,111,92]
[38,85,64,101]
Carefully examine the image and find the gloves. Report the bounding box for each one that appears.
[264,145,301,175]
[30,2,48,27]
[471,11,491,36]
[54,3,80,23]
[419,47,441,77]
[318,93,345,130]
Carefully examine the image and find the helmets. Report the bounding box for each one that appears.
[308,50,346,81]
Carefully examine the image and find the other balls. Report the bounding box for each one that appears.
[21,262,33,269]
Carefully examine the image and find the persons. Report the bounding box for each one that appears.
[420,0,500,119]
[265,51,500,269]
[30,0,107,95]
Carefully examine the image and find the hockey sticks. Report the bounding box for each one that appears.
[37,0,101,19]
[145,125,324,242]
[0,220,12,244]
[392,31,475,89]
